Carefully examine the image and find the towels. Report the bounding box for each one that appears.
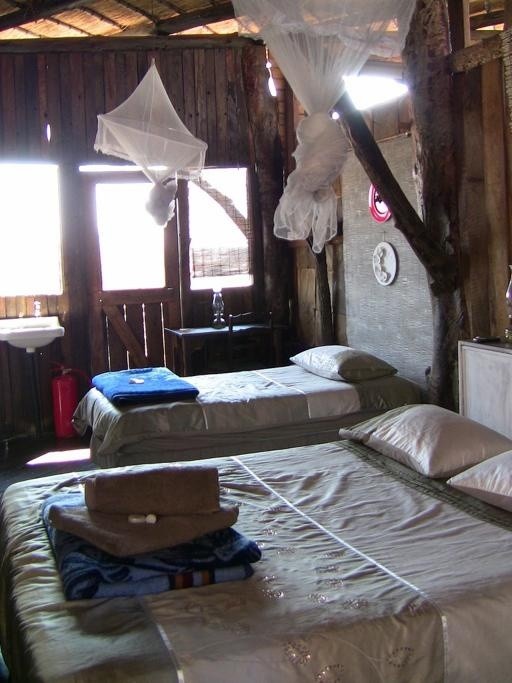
[84,465,219,515]
[48,502,239,556]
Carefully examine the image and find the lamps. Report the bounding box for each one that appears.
[269,278,296,325]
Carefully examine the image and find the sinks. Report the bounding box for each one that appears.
[0,316,65,349]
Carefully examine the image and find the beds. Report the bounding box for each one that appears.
[70,365,419,467]
[0,439,512,683]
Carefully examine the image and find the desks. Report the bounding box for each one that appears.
[165,324,289,377]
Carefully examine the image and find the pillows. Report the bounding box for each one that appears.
[446,451,512,511]
[339,402,512,479]
[289,345,398,382]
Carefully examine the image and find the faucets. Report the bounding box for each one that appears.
[34,300,41,316]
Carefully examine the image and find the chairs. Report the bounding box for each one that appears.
[201,312,266,369]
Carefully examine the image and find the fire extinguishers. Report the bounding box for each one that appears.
[49,359,90,438]
